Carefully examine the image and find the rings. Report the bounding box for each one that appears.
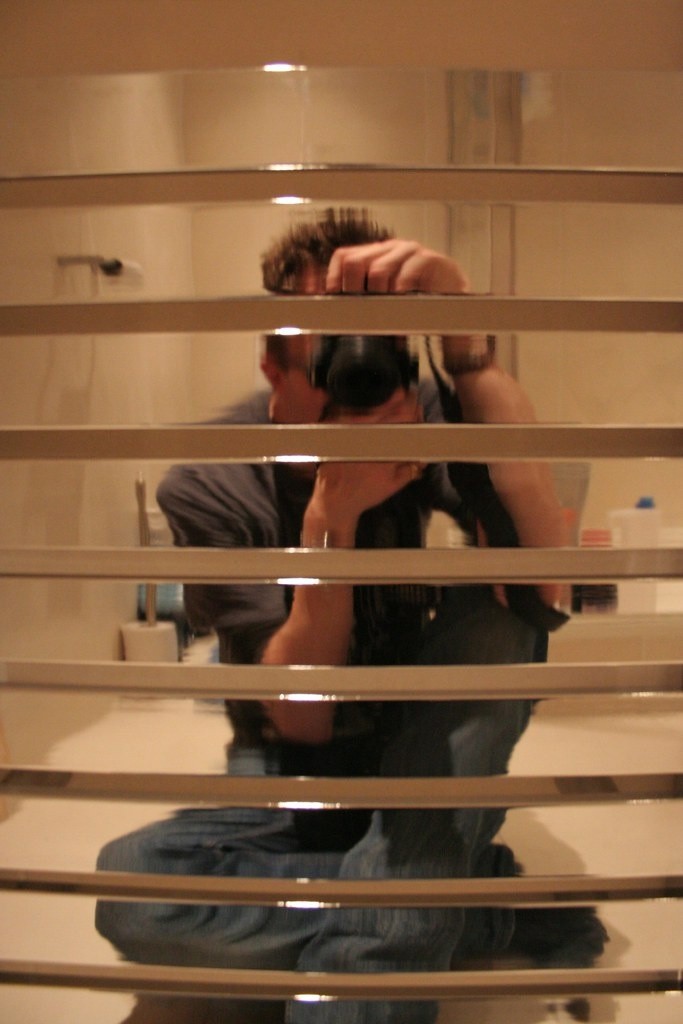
[409,462,417,480]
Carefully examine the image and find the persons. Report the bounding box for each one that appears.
[86,210,610,1024]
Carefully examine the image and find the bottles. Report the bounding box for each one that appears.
[570,528,619,614]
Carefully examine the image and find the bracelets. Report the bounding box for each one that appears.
[315,528,334,547]
[442,328,495,374]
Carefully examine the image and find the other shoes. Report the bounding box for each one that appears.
[512,909,608,967]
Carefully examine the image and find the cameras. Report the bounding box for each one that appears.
[311,335,420,416]
